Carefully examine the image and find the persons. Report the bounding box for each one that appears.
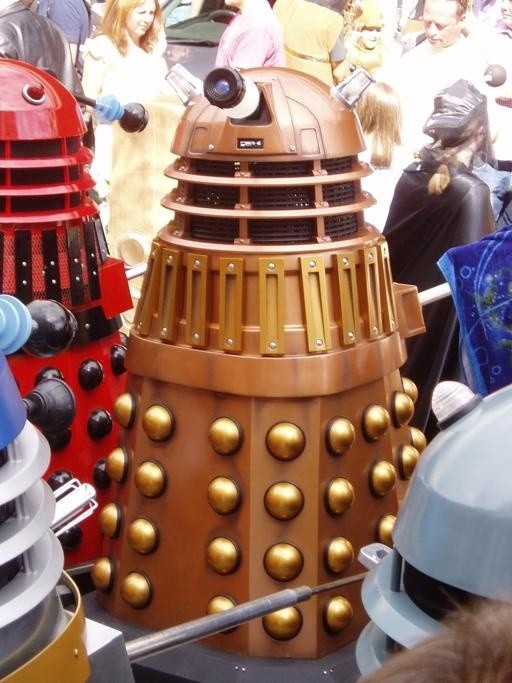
[377,77,501,452]
[356,601,511,683]
[0,0,512,235]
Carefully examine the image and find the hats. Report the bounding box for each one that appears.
[423,79,487,140]
